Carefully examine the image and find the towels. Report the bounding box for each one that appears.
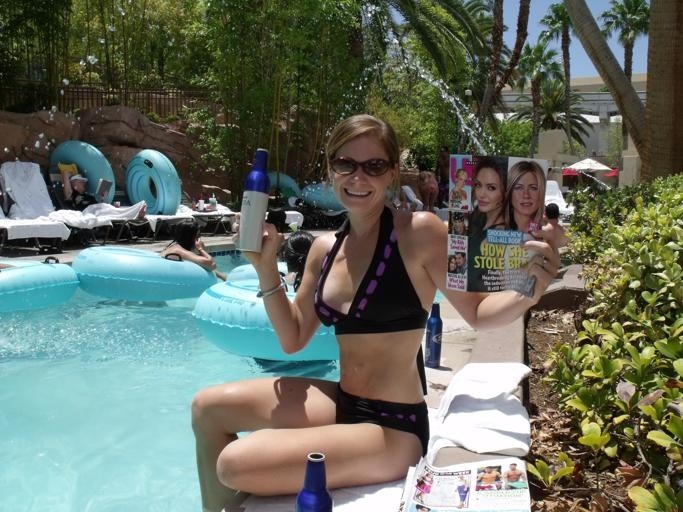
[240,476,406,512]
[427,363,530,463]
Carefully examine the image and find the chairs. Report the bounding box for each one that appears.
[0,160,235,256]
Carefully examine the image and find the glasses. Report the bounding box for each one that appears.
[328,156,395,178]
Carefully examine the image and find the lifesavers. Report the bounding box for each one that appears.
[269,169,396,210]
[73,244,217,302]
[50,140,116,204]
[126,148,182,215]
[0,257,81,314]
[193,261,340,361]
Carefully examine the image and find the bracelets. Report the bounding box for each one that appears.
[259,278,289,297]
[198,248,203,252]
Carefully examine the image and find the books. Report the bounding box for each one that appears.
[95,178,113,196]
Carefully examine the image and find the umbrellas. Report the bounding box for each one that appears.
[562,158,614,173]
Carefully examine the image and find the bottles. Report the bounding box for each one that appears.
[237,148,271,250]
[192,194,218,211]
[290,451,333,512]
[423,302,443,366]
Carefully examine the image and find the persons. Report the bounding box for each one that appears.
[435,145,452,208]
[279,232,317,287]
[543,203,569,249]
[415,474,434,503]
[419,169,438,211]
[508,161,546,233]
[448,251,467,274]
[469,158,508,291]
[477,466,501,491]
[451,169,468,199]
[191,115,559,512]
[448,212,468,235]
[455,474,470,507]
[161,219,224,279]
[505,463,526,490]
[61,169,146,221]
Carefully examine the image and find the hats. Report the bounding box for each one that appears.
[69,174,89,183]
